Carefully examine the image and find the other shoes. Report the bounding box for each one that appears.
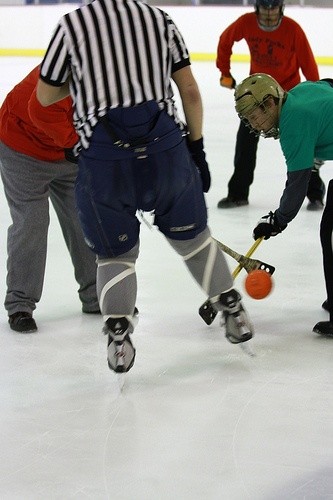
[86,306,139,317]
[307,200,324,210]
[313,321,332,336]
[8,312,37,333]
[217,197,249,209]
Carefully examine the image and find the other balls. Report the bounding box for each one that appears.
[245,271,273,300]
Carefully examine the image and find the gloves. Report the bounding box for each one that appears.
[188,137,211,192]
[253,210,284,240]
[220,74,235,89]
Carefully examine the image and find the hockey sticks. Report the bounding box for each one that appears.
[234,84,238,90]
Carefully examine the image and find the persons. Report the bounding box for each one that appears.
[35,1,254,374]
[210,1,327,212]
[0,62,139,332]
[233,72,333,342]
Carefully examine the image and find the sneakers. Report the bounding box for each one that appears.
[220,289,256,355]
[106,317,135,388]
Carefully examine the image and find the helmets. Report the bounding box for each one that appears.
[234,74,284,139]
[255,0,283,29]
